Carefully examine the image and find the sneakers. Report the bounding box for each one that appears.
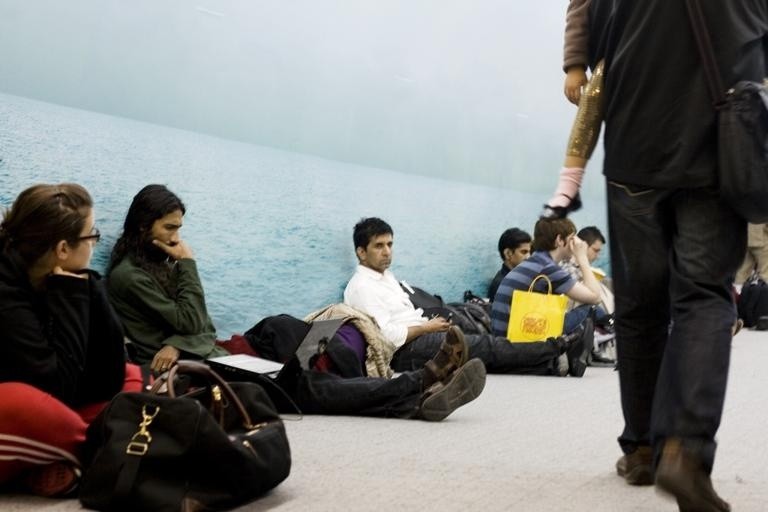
[558,305,597,378]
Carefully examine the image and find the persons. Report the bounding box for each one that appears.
[538,1,604,220]
[104,183,487,423]
[1,183,145,498]
[340,216,594,377]
[560,1,767,512]
[488,217,745,373]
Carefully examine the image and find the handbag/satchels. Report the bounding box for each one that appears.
[79,360,292,512]
[506,274,570,343]
[399,278,497,333]
[714,76,767,225]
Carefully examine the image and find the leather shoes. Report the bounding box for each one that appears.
[614,444,655,487]
[540,191,582,222]
[657,448,732,511]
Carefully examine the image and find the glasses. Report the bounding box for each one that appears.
[64,226,101,244]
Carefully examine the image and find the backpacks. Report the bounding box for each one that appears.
[738,274,768,330]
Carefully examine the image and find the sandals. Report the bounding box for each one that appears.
[416,323,468,391]
[415,357,486,423]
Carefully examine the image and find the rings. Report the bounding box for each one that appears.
[160,365,168,370]
